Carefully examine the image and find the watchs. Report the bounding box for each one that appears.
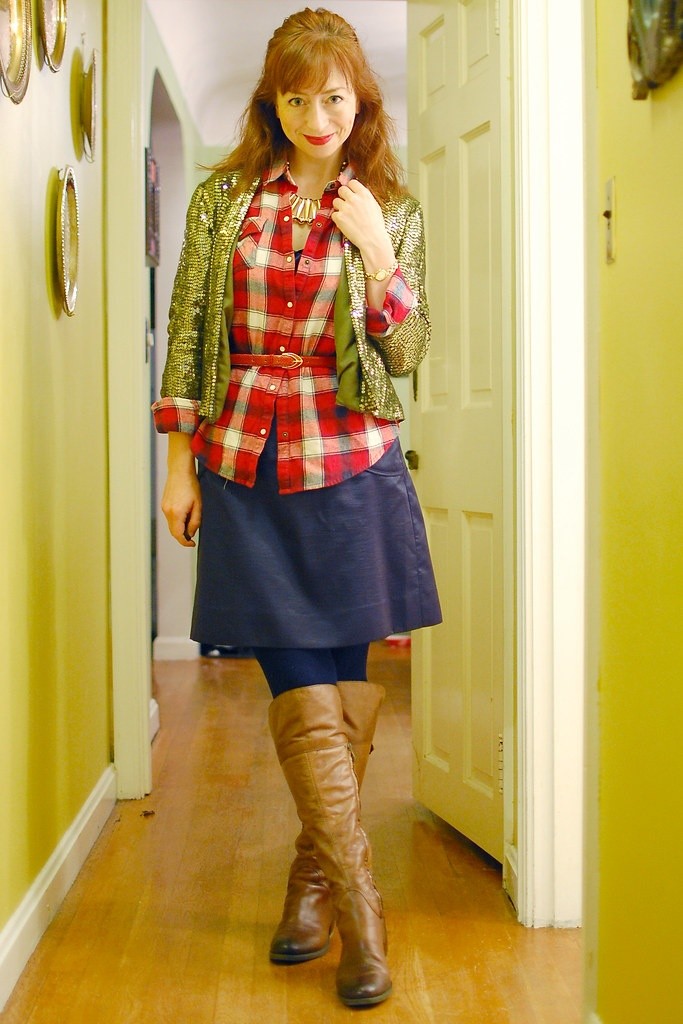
[365,260,398,282]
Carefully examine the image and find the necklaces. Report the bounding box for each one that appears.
[284,159,347,225]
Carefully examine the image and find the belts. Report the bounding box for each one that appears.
[229,353,336,370]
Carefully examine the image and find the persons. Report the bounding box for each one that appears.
[152,6,444,1009]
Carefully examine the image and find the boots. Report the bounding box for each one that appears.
[267,679,393,1007]
